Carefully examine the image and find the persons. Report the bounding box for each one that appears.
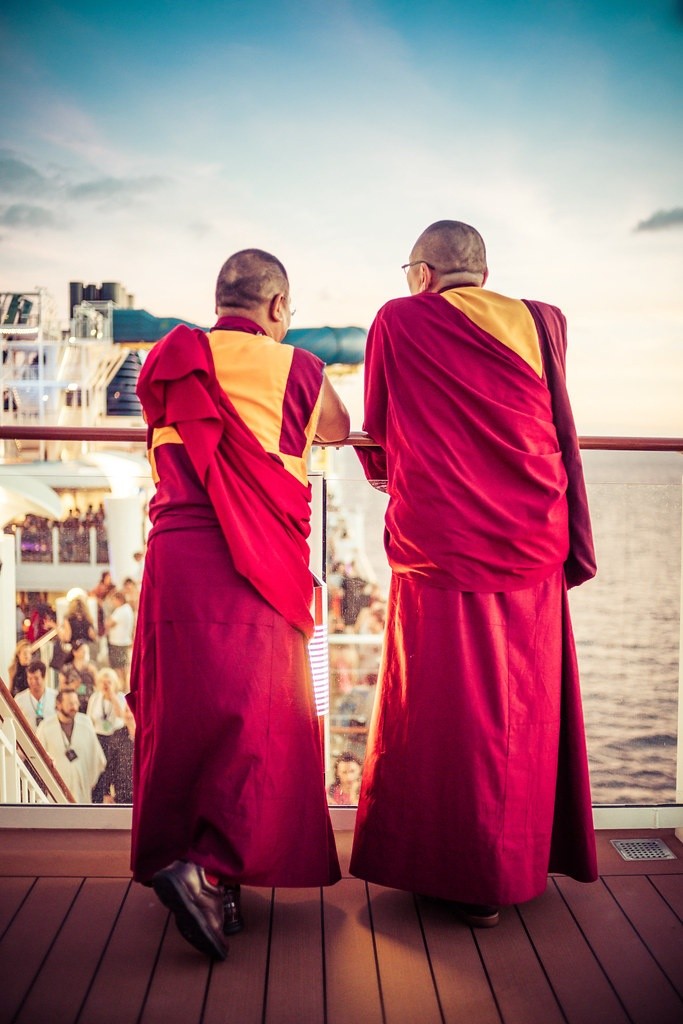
[0,489,390,806]
[134,250,351,960]
[349,220,596,926]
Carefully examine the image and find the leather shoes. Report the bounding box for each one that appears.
[150,860,227,963]
[218,879,242,936]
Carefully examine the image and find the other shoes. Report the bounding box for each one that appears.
[451,896,501,929]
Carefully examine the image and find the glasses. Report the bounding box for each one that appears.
[401,260,435,275]
